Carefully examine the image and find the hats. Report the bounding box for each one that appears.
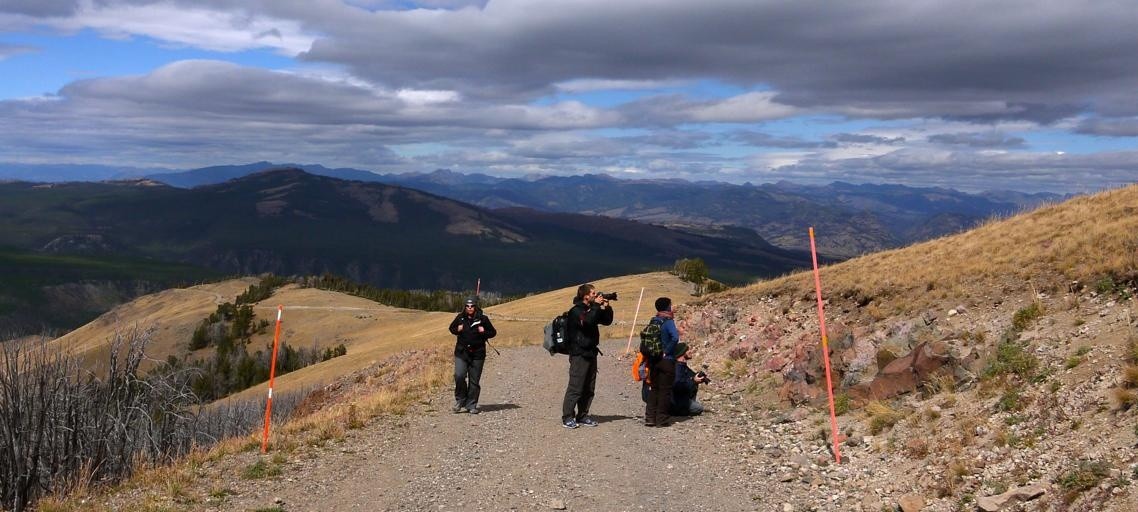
[674,342,689,359]
[654,297,671,312]
[464,297,478,306]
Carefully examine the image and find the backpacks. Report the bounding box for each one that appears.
[551,313,575,355]
[640,316,672,363]
[631,351,654,404]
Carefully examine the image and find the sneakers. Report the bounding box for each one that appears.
[575,415,599,427]
[561,418,581,429]
[452,402,479,414]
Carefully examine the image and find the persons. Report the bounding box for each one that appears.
[448,294,497,416]
[672,342,707,416]
[560,283,615,431]
[638,296,678,429]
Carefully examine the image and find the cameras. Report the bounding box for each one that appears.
[597,292,618,301]
[698,371,712,384]
